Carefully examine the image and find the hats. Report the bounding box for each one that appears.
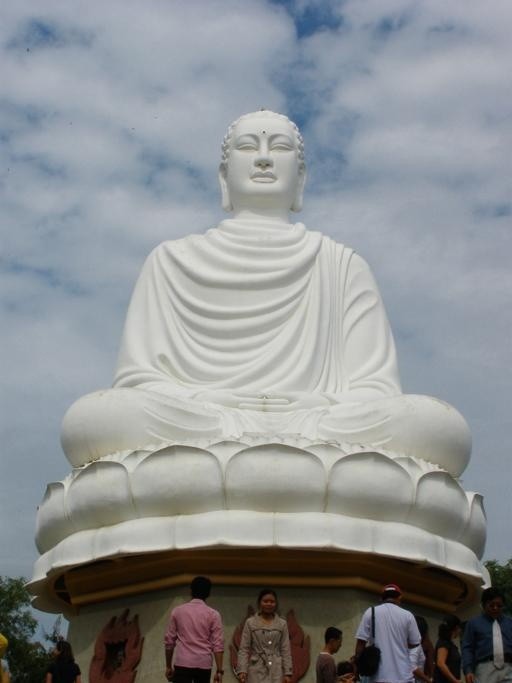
[380,584,401,594]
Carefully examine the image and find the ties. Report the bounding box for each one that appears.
[493,619,504,670]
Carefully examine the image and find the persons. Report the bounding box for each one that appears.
[44,641,81,683]
[0,634,10,683]
[60,109,471,479]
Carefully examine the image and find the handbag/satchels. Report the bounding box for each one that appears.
[357,645,380,676]
